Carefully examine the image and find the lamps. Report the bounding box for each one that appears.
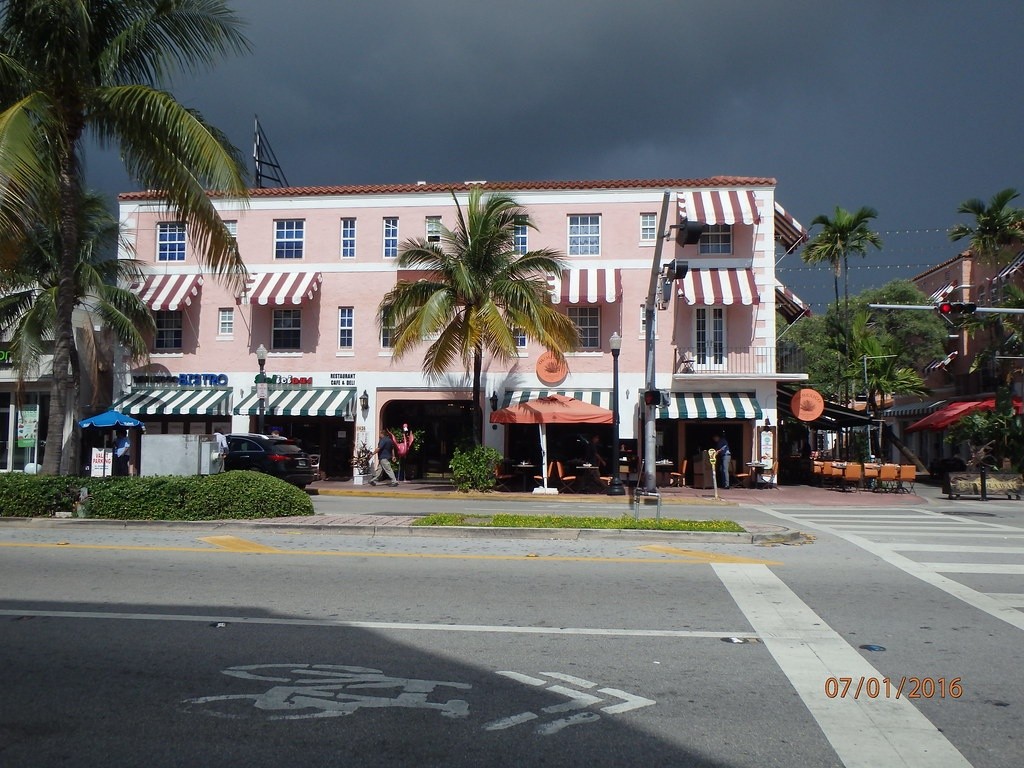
[489,390,498,412]
[360,390,368,410]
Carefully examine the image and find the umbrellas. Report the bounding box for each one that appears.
[78,410,143,478]
[490,394,613,495]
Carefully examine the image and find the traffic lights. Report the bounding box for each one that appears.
[644,390,661,404]
[938,302,976,328]
[667,259,688,279]
[676,218,703,248]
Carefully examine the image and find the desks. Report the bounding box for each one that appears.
[575,466,599,493]
[512,464,534,492]
[655,464,674,487]
[745,463,766,487]
[938,471,1023,500]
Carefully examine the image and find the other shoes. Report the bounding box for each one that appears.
[578,487,588,494]
[389,482,399,487]
[600,486,609,494]
[368,481,376,486]
[718,484,729,489]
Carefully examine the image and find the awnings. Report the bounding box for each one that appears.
[777,386,872,461]
[905,398,1024,433]
[775,277,812,340]
[926,281,955,304]
[546,268,623,338]
[127,274,204,347]
[923,351,957,380]
[675,191,760,268]
[637,389,762,419]
[993,248,1024,284]
[107,387,234,416]
[234,271,322,335]
[774,201,809,267]
[883,399,954,417]
[234,385,358,417]
[671,268,760,342]
[501,390,613,410]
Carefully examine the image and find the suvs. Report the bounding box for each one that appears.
[220,433,314,488]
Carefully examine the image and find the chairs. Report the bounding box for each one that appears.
[730,459,779,490]
[534,461,553,486]
[556,462,576,494]
[493,465,512,492]
[598,464,618,495]
[669,460,688,488]
[810,453,916,495]
[678,351,696,374]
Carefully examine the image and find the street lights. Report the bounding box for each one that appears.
[256,344,268,434]
[608,333,627,495]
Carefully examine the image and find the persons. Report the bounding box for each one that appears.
[112,428,131,476]
[713,434,732,489]
[368,429,399,487]
[779,436,811,484]
[213,426,230,473]
[272,430,279,436]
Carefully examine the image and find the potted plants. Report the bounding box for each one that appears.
[350,442,373,486]
